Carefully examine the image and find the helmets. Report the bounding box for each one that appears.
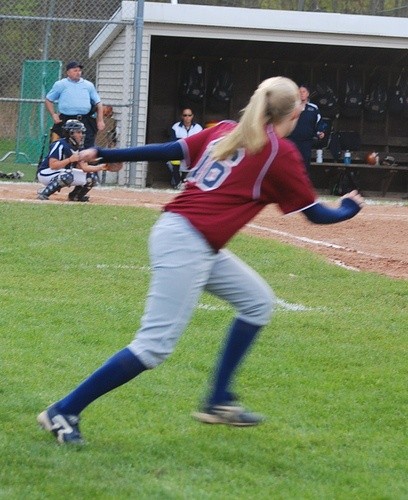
[60,118,84,135]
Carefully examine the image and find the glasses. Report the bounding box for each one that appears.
[182,114,193,117]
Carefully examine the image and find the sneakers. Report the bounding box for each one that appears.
[36,402,82,451]
[194,394,264,426]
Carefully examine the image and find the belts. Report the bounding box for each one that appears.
[62,115,88,121]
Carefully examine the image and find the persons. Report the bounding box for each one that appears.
[284,83,324,178]
[36,119,111,202]
[44,62,106,187]
[164,107,204,188]
[37,75,366,451]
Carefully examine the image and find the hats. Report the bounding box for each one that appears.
[66,62,84,71]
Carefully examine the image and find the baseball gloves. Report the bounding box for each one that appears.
[105,161,122,172]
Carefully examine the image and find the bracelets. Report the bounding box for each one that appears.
[68,158,71,163]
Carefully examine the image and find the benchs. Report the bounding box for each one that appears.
[308,131,408,199]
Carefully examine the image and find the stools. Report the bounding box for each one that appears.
[170,159,189,189]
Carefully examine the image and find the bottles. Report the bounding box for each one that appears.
[344,150,351,163]
[316,147,323,163]
